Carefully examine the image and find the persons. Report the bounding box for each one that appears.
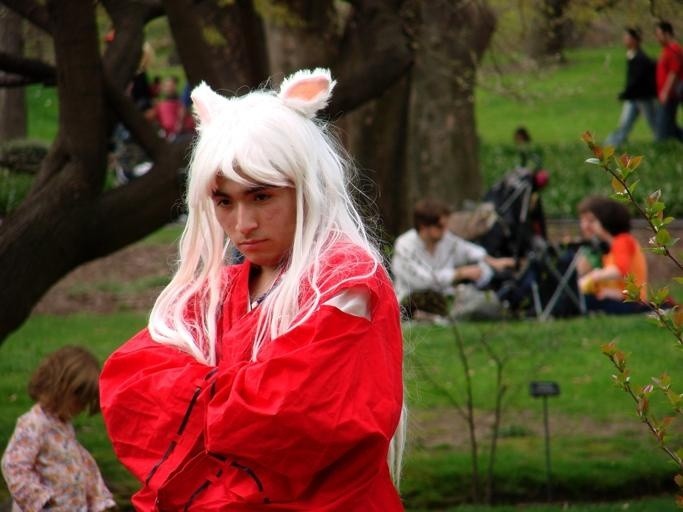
[96,64,409,512]
[654,20,683,142]
[527,195,648,320]
[97,28,196,191]
[0,345,117,512]
[513,129,530,144]
[388,196,527,327]
[603,26,657,149]
